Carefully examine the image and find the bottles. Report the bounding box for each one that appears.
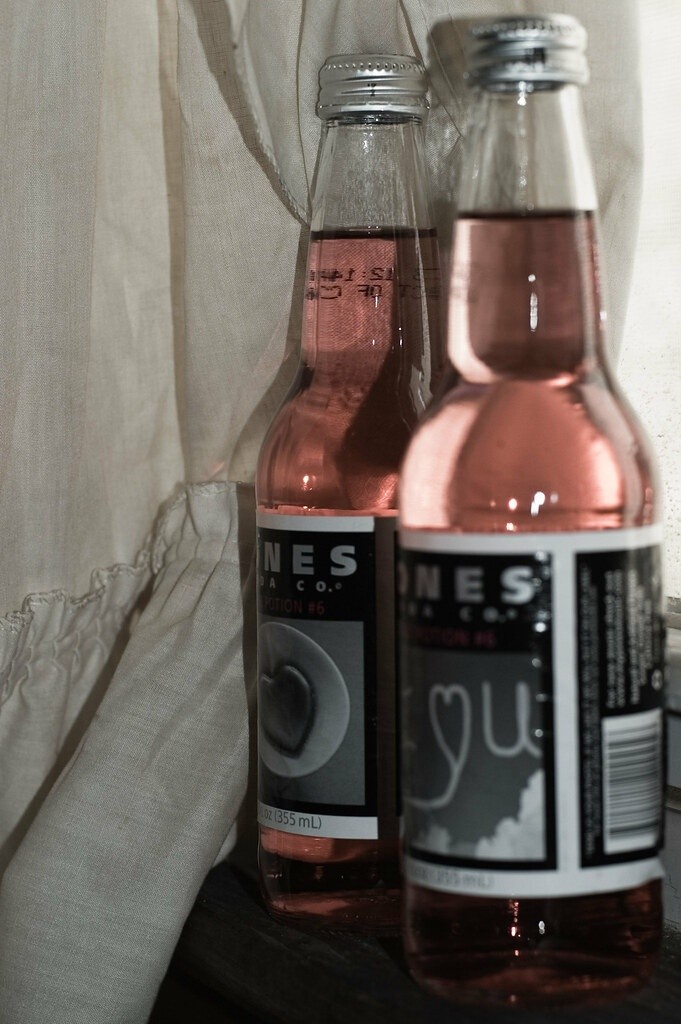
[250,50,459,938]
[392,12,672,1011]
